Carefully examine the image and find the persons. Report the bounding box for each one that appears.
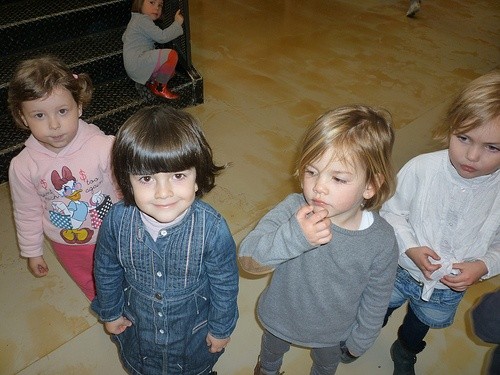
[237,106,399,375]
[121,0,184,101]
[9,54,116,299]
[339,0,500,375]
[89,107,239,375]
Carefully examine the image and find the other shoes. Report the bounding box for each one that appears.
[406,2,421,17]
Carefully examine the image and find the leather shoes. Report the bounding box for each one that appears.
[150,79,179,100]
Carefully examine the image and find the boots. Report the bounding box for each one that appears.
[389,325,426,375]
